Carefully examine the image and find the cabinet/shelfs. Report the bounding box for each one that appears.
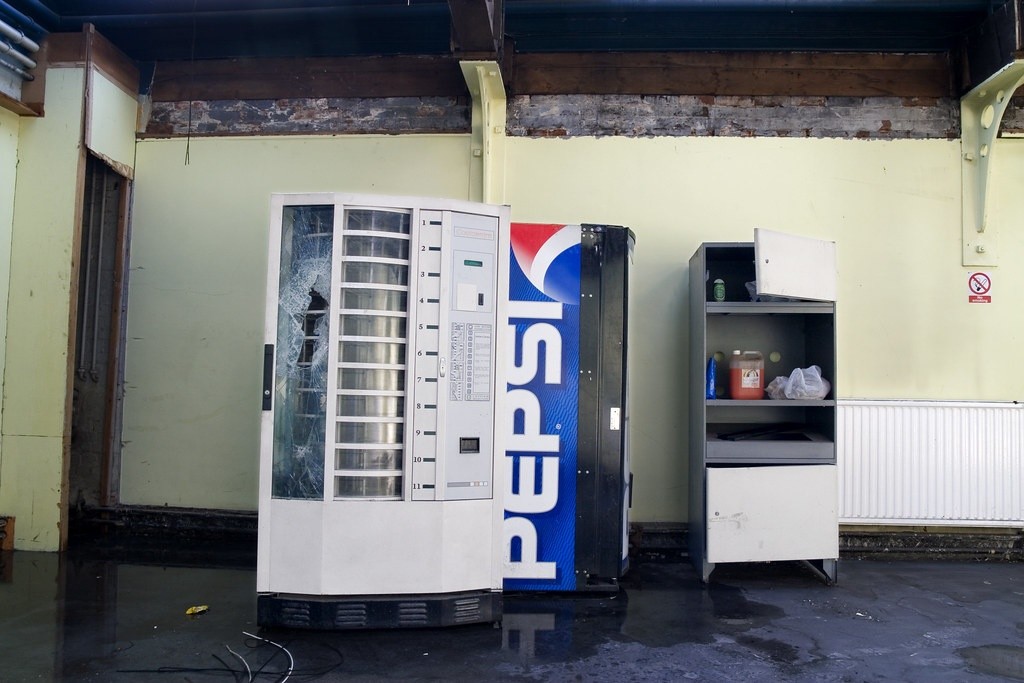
[686,225,843,590]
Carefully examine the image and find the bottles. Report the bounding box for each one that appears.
[731,351,764,399]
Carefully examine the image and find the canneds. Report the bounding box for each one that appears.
[713,279,725,302]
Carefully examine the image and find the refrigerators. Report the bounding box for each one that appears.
[504,222,636,591]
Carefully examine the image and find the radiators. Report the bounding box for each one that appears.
[835,399,1024,529]
